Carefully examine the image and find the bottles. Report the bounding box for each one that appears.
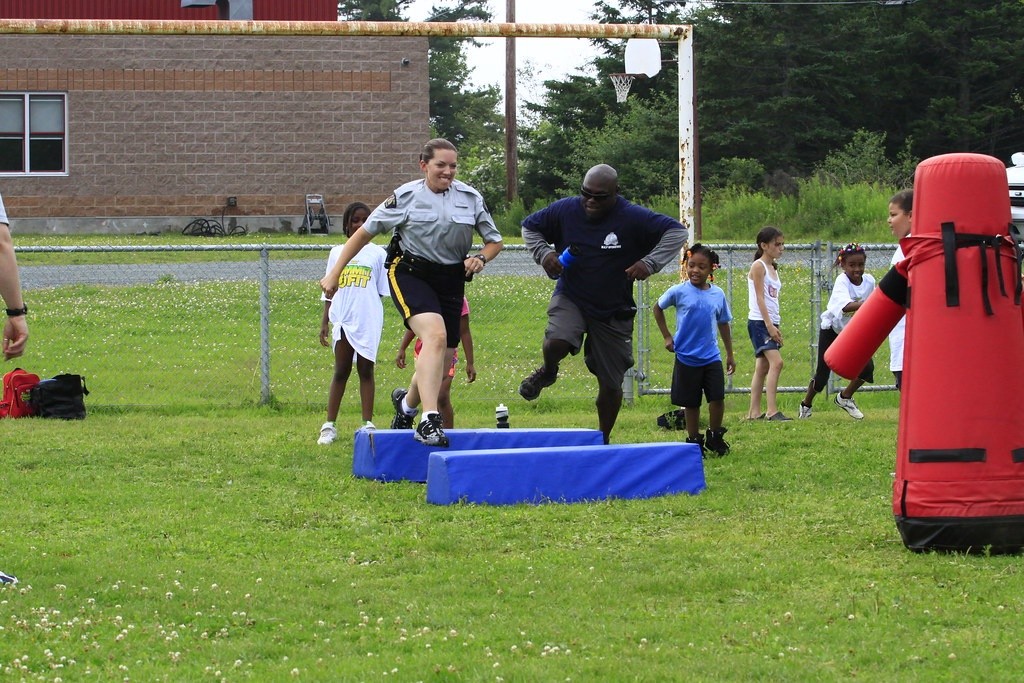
[547,243,581,280]
[496,403,509,428]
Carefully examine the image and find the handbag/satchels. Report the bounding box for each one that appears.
[30,374,89,420]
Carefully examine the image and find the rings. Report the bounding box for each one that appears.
[475,267,478,270]
[3,338,8,341]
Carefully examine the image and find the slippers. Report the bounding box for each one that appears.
[768,411,794,422]
[757,413,766,419]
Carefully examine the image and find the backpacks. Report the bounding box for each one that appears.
[0,368,40,418]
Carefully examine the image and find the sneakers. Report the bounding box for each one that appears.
[414,413,449,447]
[705,427,731,456]
[361,421,375,430]
[686,434,707,459]
[317,422,337,445]
[833,391,864,419]
[798,399,812,418]
[519,367,557,400]
[390,388,418,429]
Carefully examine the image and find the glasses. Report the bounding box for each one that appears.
[580,184,618,201]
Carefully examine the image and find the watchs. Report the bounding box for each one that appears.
[475,254,486,264]
[5,303,28,316]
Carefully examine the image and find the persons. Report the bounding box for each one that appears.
[886,188,914,477]
[0,193,29,584]
[798,243,876,419]
[317,201,392,445]
[746,227,795,422]
[516,163,689,446]
[653,243,735,459]
[320,138,504,447]
[396,295,477,429]
